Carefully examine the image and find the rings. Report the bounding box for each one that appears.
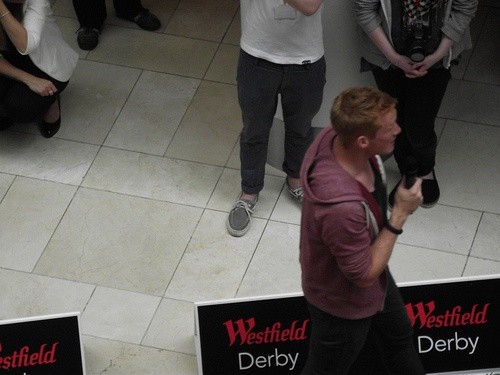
[49,90,52,94]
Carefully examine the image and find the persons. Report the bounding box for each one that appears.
[0,0,79,138]
[226,0,327,236]
[352,0,479,213]
[72,0,162,50]
[299,87,423,375]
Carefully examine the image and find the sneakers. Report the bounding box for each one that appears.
[227,190,259,237]
[285,175,304,208]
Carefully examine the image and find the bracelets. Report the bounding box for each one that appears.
[383,219,403,235]
[0,11,10,18]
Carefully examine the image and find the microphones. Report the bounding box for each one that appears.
[403,157,417,189]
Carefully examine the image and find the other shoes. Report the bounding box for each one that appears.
[0,115,13,132]
[38,94,61,139]
[74,18,105,51]
[115,6,161,31]
[388,164,440,211]
